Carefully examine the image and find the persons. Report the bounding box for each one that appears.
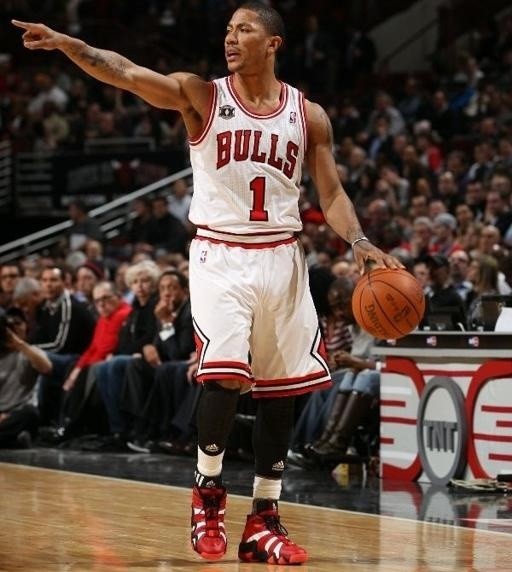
[2,1,512,469]
[13,0,406,564]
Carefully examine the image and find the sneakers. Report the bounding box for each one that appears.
[191,482,227,560]
[238,513,307,565]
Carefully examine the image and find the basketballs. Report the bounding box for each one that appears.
[352,267,425,338]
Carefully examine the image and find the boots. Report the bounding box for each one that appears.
[309,390,373,458]
[304,391,350,456]
